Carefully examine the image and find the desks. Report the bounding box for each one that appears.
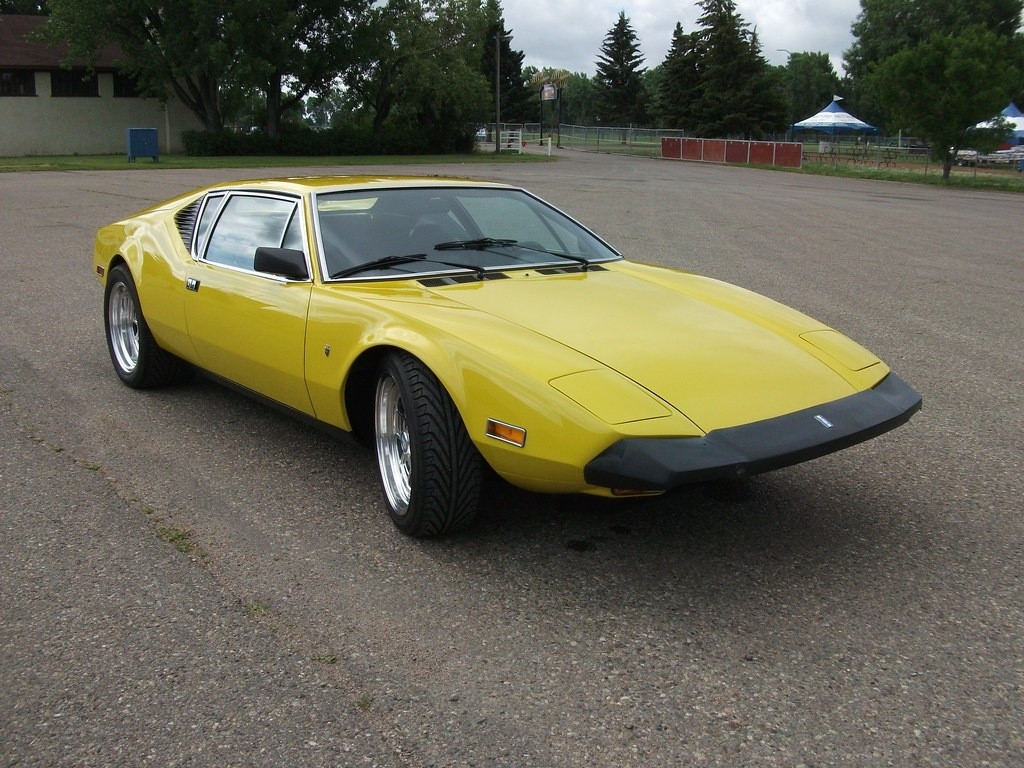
[822,145,931,168]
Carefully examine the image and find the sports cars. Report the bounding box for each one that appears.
[88,170,924,540]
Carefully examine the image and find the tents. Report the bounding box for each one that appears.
[790,101,879,154]
[966,102,1024,145]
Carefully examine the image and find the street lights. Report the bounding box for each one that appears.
[492,32,514,152]
[778,48,797,142]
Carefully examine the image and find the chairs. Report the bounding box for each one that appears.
[408,224,457,252]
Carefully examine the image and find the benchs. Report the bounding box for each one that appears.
[802,151,925,168]
